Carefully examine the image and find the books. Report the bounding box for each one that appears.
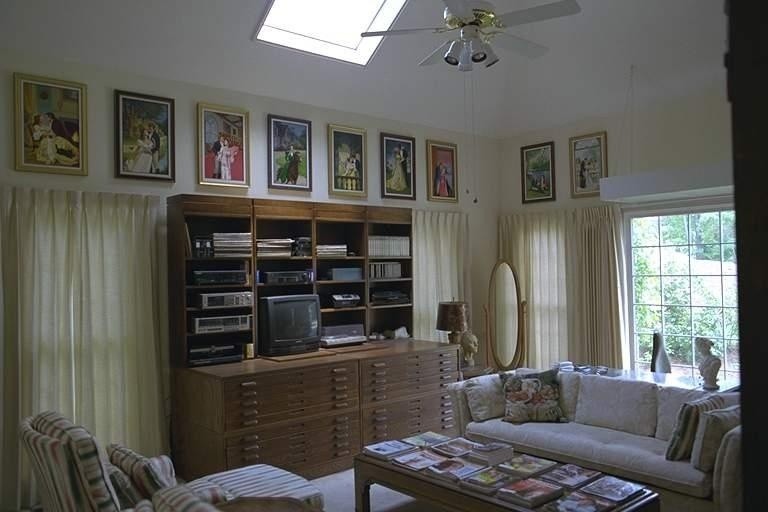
[544,490,617,512]
[425,456,491,483]
[368,236,410,257]
[214,233,253,258]
[316,244,347,258]
[364,438,419,462]
[494,454,558,478]
[469,442,514,466]
[432,437,485,457]
[497,477,564,508]
[401,431,451,451]
[458,467,522,494]
[571,475,648,507]
[256,239,295,258]
[390,449,450,472]
[541,464,601,489]
[369,261,402,279]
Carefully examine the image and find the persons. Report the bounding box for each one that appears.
[127,123,161,175]
[211,132,234,180]
[342,155,360,191]
[277,144,301,186]
[579,158,594,189]
[277,145,298,184]
[31,111,80,166]
[529,174,549,193]
[694,336,721,389]
[386,144,408,191]
[462,333,479,366]
[434,161,449,198]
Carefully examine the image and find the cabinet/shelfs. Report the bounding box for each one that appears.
[165,193,461,483]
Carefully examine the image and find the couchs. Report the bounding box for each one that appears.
[18,409,324,512]
[447,367,744,512]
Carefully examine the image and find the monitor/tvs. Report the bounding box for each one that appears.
[258,293,322,358]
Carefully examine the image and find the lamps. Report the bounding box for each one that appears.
[458,48,474,72]
[443,40,459,66]
[485,43,501,68]
[468,37,489,64]
[436,300,469,344]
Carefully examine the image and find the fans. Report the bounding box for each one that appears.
[361,0,581,67]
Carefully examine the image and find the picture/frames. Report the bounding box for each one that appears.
[266,112,313,192]
[426,139,459,203]
[568,129,609,200]
[381,132,417,201]
[520,141,556,203]
[326,122,368,199]
[195,101,252,190]
[113,88,177,184]
[13,70,89,177]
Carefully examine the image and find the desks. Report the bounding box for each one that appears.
[574,365,741,393]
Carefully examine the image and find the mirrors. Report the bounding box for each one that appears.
[482,257,528,371]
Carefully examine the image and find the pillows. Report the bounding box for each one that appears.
[106,439,168,498]
[690,404,741,472]
[664,395,726,461]
[101,461,142,504]
[463,374,506,421]
[501,366,566,423]
